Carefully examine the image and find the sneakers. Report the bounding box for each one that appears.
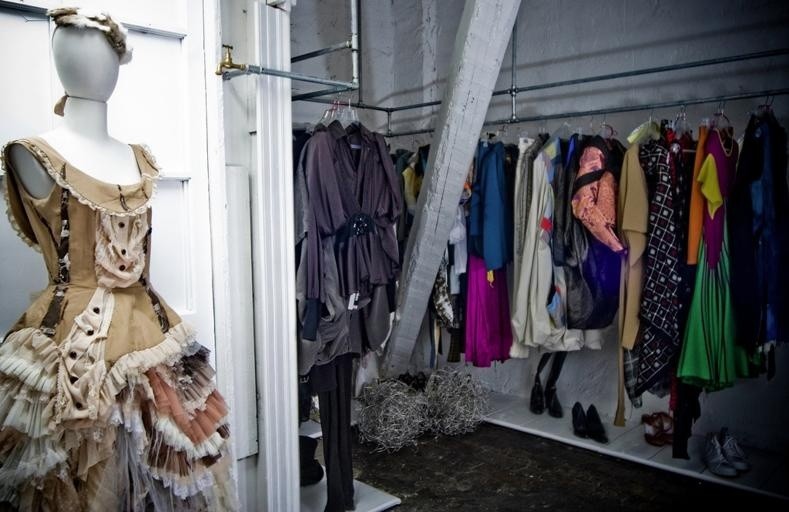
[699,430,737,479]
[718,425,751,473]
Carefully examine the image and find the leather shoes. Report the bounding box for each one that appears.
[530,382,547,417]
[585,402,609,445]
[641,411,674,448]
[571,401,590,439]
[544,382,564,419]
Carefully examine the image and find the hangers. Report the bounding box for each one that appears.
[626,99,693,160]
[387,129,433,154]
[699,95,733,145]
[481,108,619,151]
[738,91,776,145]
[311,96,366,143]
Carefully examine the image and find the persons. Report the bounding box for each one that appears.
[0,6,239,512]
